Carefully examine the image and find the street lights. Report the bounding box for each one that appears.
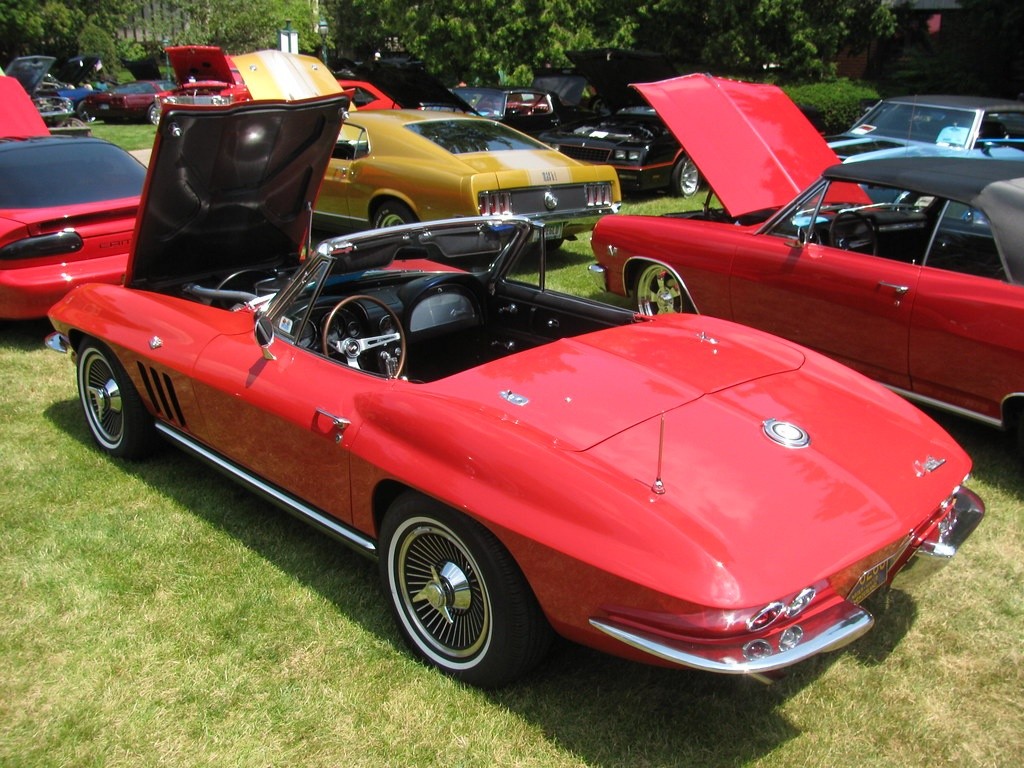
[318,18,329,66]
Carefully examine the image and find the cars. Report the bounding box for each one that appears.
[822,94,1024,223]
[231,51,622,267]
[46,86,986,689]
[86,80,178,125]
[0,66,148,318]
[148,43,253,127]
[535,49,702,199]
[584,71,1024,463]
[336,79,402,110]
[53,55,108,124]
[6,55,77,125]
[349,50,589,136]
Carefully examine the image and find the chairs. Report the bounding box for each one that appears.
[980,121,1007,139]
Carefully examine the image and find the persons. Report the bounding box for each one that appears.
[82,79,93,91]
[67,81,76,90]
[483,99,503,116]
